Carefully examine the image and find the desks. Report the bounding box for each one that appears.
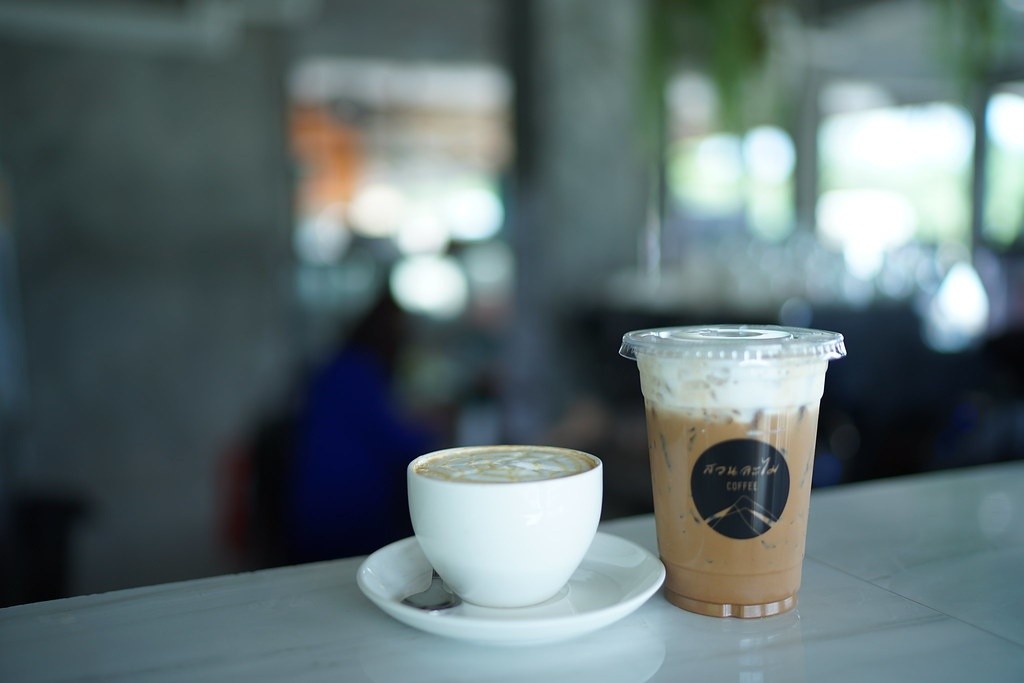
[0,462,1024,683]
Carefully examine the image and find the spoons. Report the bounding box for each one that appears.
[400,568,462,610]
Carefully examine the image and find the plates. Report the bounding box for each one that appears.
[356,533,667,647]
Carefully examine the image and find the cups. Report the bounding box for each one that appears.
[618,324,848,620]
[407,445,603,610]
